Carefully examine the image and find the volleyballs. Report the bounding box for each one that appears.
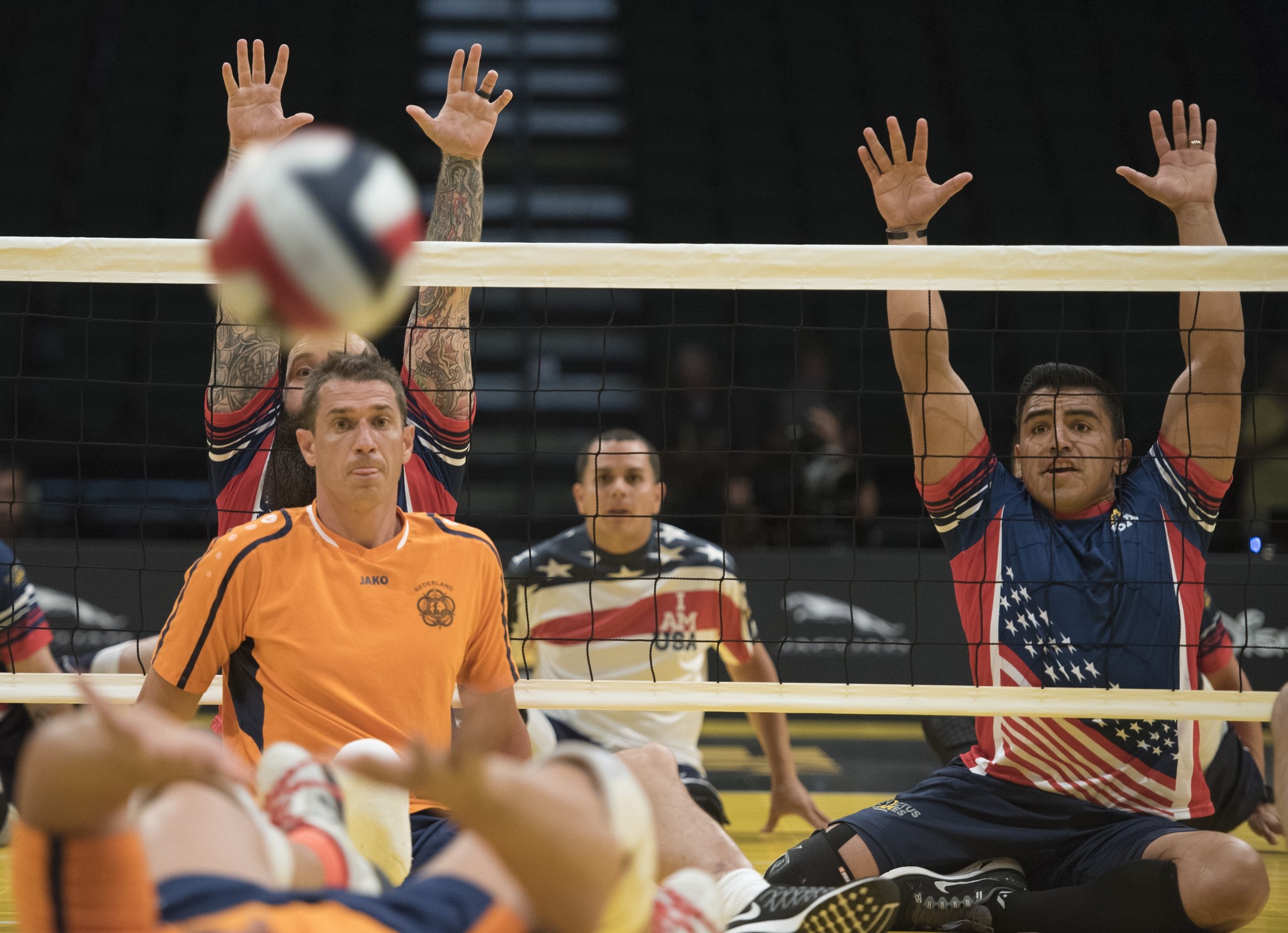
[208,132,423,341]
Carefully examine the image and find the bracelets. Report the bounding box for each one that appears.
[885,225,927,239]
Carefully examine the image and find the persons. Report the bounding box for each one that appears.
[755,100,1281,932]
[489,426,835,854]
[0,345,1287,931]
[203,27,513,795]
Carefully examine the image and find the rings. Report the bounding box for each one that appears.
[1189,139,1203,148]
[477,89,491,99]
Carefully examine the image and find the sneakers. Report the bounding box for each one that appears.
[876,857,1027,932]
[255,740,381,899]
[720,875,901,932]
[656,866,725,933]
